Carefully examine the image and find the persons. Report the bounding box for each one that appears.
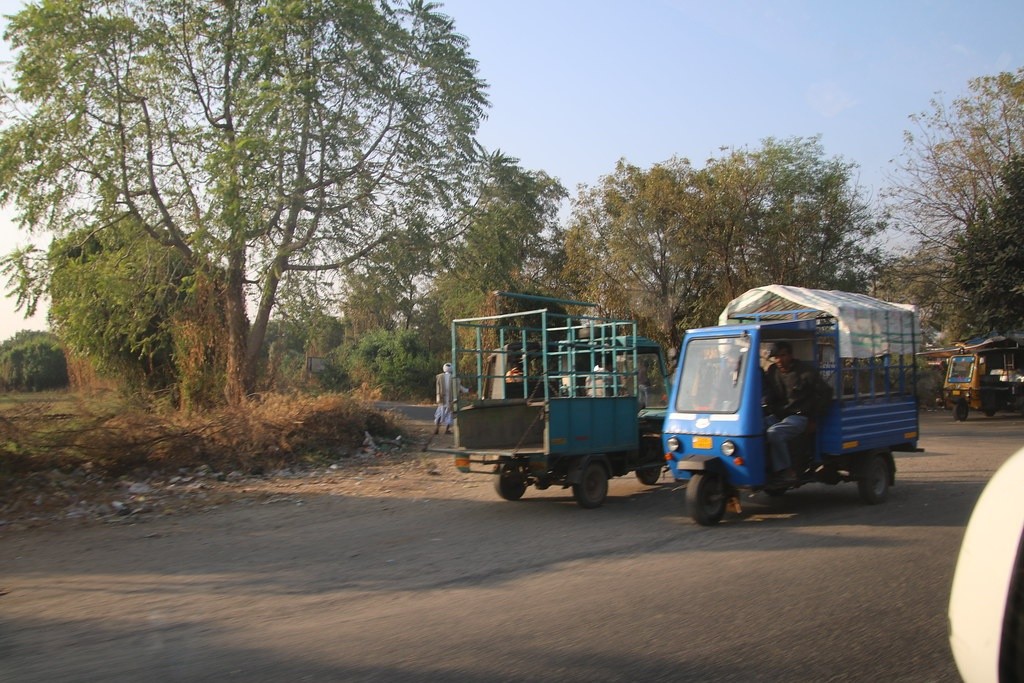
[506,356,523,381]
[434,363,472,434]
[585,354,620,396]
[715,341,834,482]
[999,363,1024,382]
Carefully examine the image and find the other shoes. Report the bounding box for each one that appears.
[782,469,797,482]
[434,430,438,434]
[446,430,453,434]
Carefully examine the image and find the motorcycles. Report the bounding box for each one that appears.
[660,281,928,528]
[940,348,1024,423]
[418,290,674,509]
